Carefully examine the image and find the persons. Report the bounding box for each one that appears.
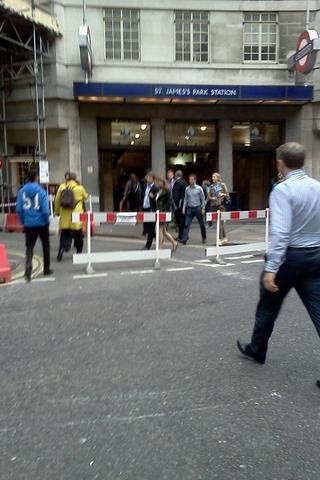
[141,172,178,254]
[172,170,189,241]
[208,173,229,246]
[53,172,88,261]
[16,169,54,280]
[122,174,141,212]
[202,180,213,227]
[179,174,206,245]
[236,142,320,387]
[167,170,175,190]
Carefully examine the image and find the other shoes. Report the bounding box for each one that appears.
[218,238,228,246]
[201,239,206,243]
[236,337,266,364]
[172,242,179,252]
[57,248,64,261]
[24,272,32,283]
[43,269,54,276]
[178,238,187,246]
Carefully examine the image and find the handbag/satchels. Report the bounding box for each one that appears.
[223,197,232,208]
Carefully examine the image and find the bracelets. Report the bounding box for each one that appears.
[202,207,205,209]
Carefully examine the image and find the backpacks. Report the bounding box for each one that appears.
[58,185,81,210]
